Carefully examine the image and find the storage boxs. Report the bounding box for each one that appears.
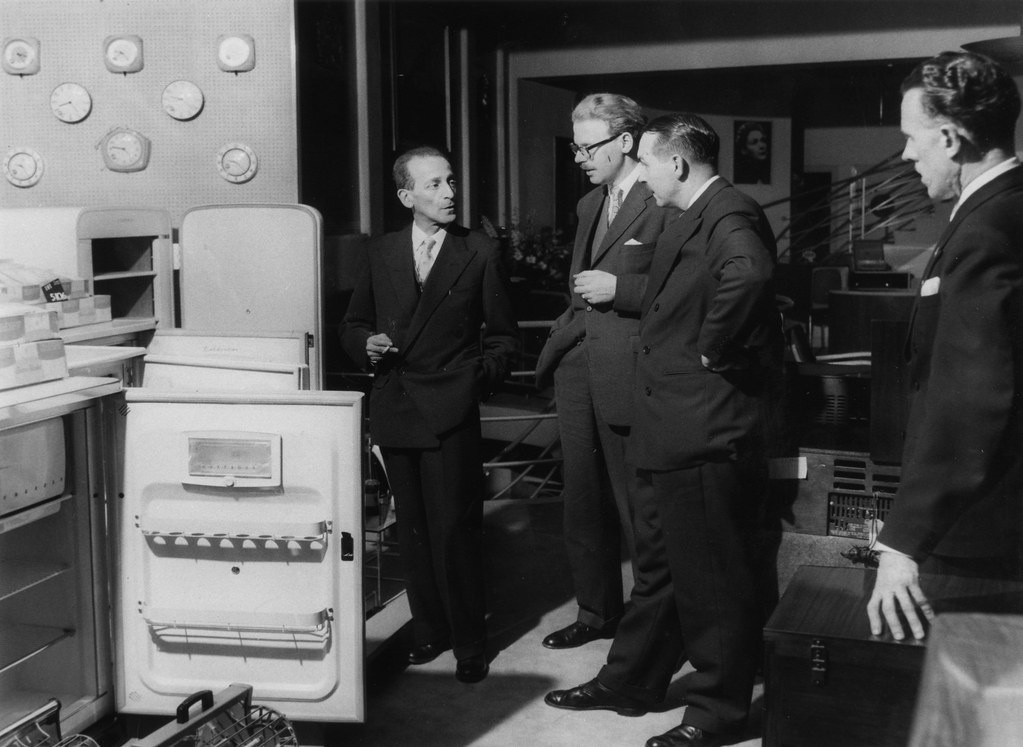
[760,565,929,747]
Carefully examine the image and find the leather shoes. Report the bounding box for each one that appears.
[404,639,451,664]
[545,679,650,717]
[542,620,615,649]
[647,724,747,747]
[456,651,488,683]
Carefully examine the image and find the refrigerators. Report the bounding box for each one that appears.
[0,200,365,739]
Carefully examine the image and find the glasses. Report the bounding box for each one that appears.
[571,133,623,160]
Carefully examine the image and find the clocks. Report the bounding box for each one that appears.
[49,83,92,123]
[162,79,205,121]
[215,32,255,76]
[104,35,144,75]
[0,36,40,77]
[97,125,150,172]
[3,147,44,187]
[217,143,257,182]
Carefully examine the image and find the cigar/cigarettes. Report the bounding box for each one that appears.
[382,345,390,355]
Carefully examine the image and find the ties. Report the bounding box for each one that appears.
[417,238,435,285]
[607,187,625,228]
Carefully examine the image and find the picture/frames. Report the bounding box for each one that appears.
[733,119,773,185]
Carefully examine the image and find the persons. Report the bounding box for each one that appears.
[537,93,684,650]
[337,147,517,686]
[868,49,1023,640]
[544,114,770,747]
